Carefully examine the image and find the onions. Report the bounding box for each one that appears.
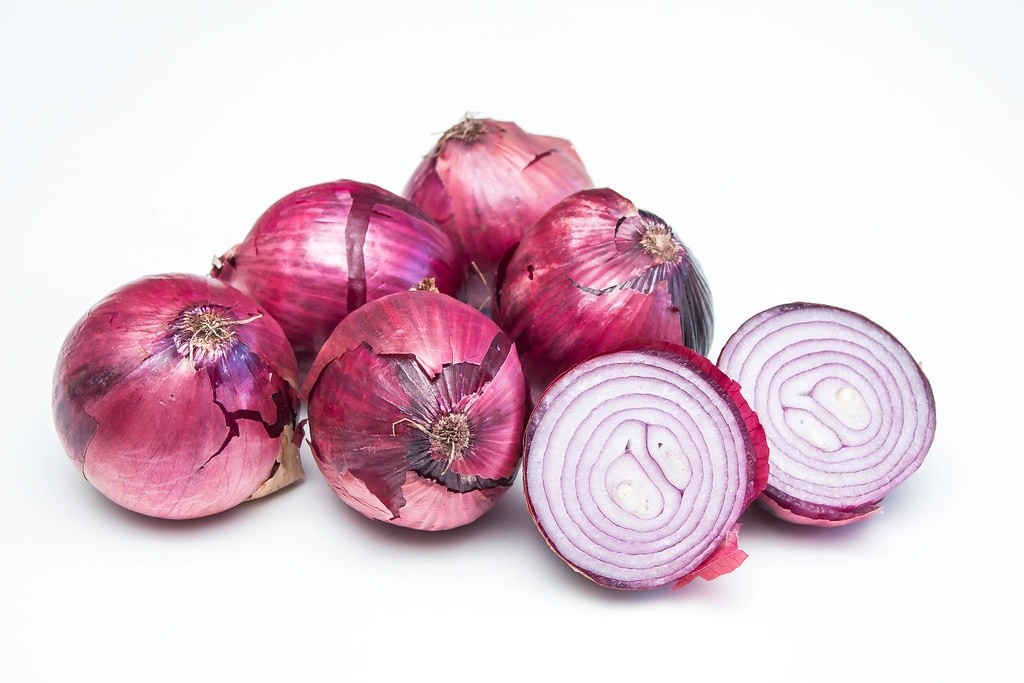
[53,118,936,591]
